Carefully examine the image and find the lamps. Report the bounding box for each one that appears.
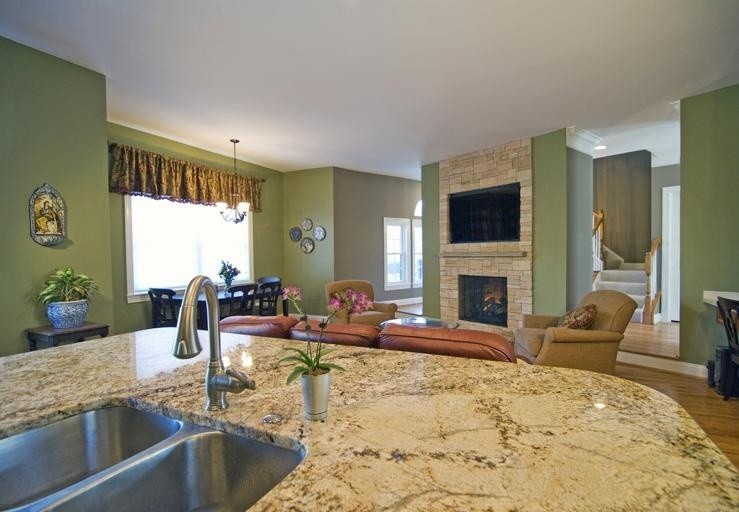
[219,137,249,224]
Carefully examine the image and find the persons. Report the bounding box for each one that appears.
[36,201,56,231]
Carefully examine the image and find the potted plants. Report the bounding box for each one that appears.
[37,264,100,329]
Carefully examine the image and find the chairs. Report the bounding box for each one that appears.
[147,288,200,329]
[226,283,258,316]
[260,280,283,316]
[255,276,283,314]
[713,295,738,401]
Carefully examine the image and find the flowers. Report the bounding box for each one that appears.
[279,283,374,385]
[217,259,241,281]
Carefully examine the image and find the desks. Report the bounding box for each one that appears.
[162,289,289,329]
[702,290,739,330]
[26,320,110,350]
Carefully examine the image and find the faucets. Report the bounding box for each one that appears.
[172,275,256,412]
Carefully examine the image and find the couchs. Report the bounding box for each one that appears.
[219,316,516,363]
[325,278,398,324]
[514,288,639,376]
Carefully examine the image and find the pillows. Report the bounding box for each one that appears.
[556,303,599,331]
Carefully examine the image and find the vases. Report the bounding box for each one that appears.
[298,367,332,419]
[223,280,234,295]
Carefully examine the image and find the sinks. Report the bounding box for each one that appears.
[0,396,183,511]
[40,423,307,510]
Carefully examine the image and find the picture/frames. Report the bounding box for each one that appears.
[29,183,66,248]
